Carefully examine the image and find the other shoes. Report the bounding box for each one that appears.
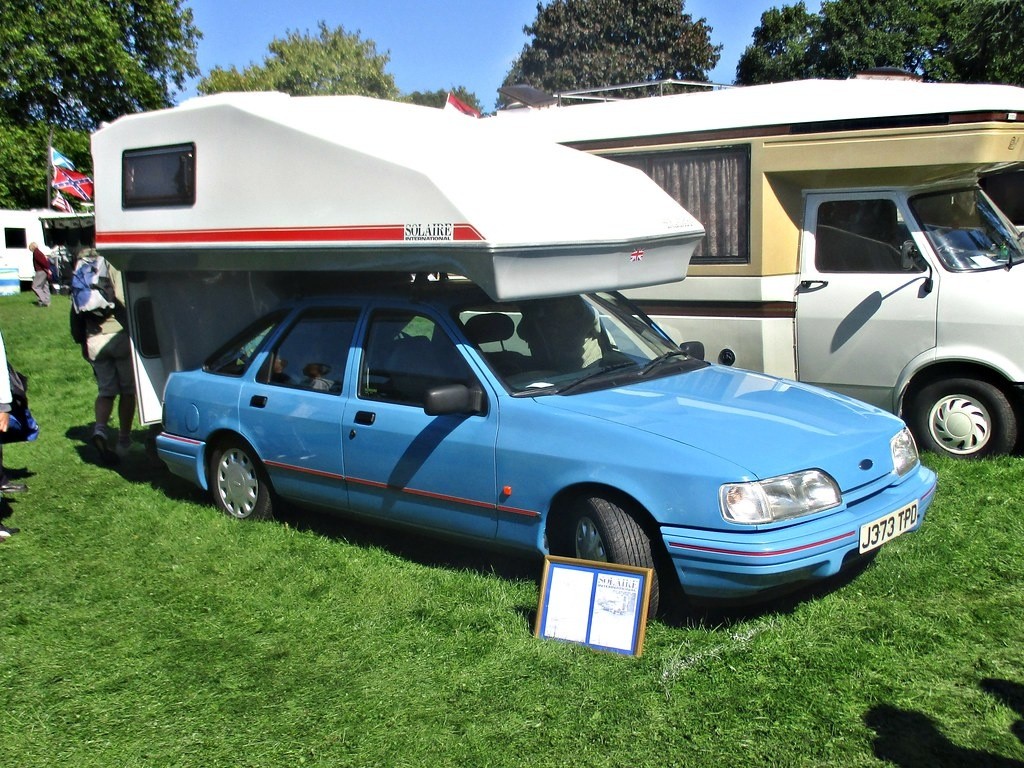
[0,523,20,537]
[115,438,145,457]
[0,473,29,494]
[91,431,121,465]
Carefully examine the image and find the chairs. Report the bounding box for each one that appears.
[465,313,530,377]
[383,337,448,404]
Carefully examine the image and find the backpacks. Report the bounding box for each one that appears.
[0,360,39,444]
[71,254,116,315]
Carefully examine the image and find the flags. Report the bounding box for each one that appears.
[48,146,94,213]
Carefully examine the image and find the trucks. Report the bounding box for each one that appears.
[507,75,1024,463]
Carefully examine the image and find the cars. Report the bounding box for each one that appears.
[154,276,937,625]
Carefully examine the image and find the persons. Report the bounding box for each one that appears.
[273,356,341,394]
[29,241,51,308]
[69,260,147,455]
[516,293,601,368]
[0,330,28,534]
